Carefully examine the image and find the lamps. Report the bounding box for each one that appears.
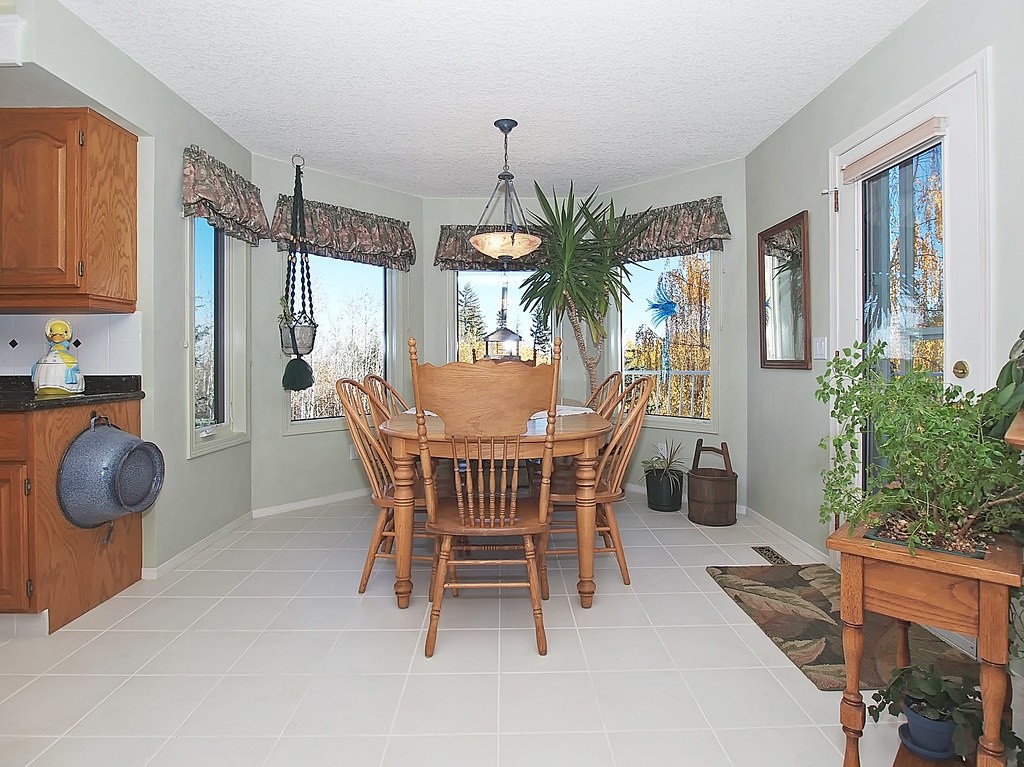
[468,117,542,270]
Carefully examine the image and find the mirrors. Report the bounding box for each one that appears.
[757,210,813,372]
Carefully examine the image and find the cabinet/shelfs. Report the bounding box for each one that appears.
[0,398,143,635]
[0,107,138,315]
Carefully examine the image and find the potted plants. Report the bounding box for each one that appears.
[867,661,1024,767]
[274,298,317,355]
[525,178,658,485]
[816,340,989,561]
[640,439,690,513]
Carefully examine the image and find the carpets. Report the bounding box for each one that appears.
[706,563,982,691]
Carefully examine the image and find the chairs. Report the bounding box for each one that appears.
[334,337,654,659]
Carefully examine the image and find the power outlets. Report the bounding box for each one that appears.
[657,444,668,460]
[349,444,360,460]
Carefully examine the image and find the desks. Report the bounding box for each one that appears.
[379,397,614,612]
[827,508,1024,767]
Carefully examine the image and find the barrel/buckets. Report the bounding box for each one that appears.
[687,437,738,527]
[56,416,165,544]
